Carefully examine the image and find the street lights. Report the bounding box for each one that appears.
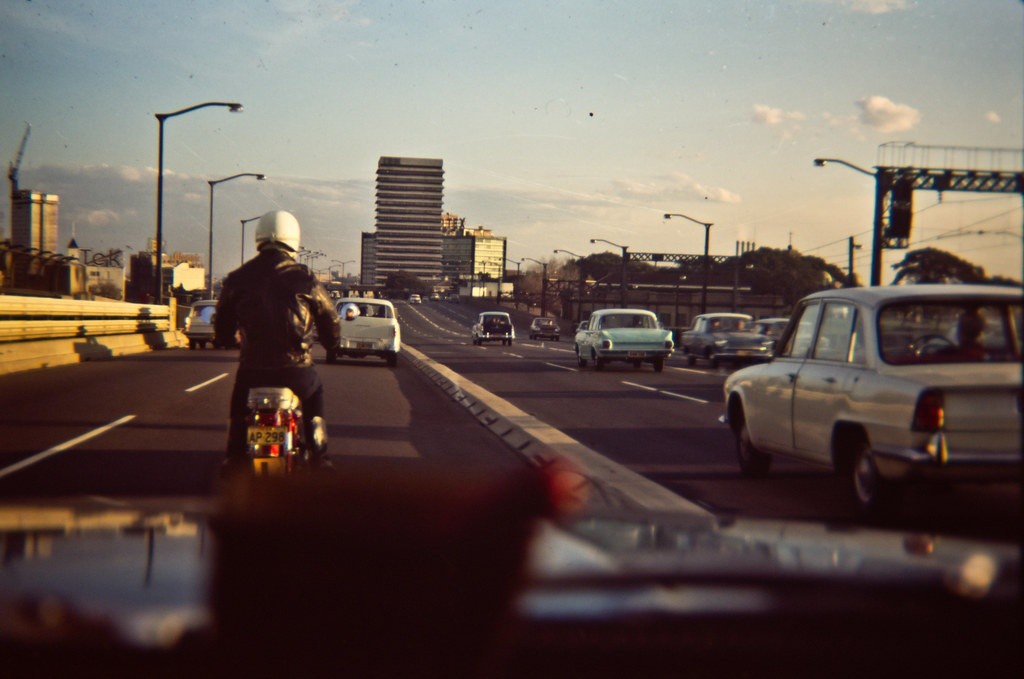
[521,257,548,319]
[499,257,521,311]
[812,156,882,289]
[662,212,714,315]
[553,249,586,322]
[153,100,246,305]
[589,239,630,308]
[205,172,267,301]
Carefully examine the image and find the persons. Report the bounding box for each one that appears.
[213,208,340,478]
[627,316,642,327]
[936,312,994,355]
[343,303,357,321]
[734,318,746,331]
[708,319,723,332]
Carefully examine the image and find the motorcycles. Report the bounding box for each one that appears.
[238,389,319,505]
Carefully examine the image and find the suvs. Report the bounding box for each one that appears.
[186,301,241,349]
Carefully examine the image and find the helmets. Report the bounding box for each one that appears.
[255,210,302,258]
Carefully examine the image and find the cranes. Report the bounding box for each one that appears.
[4,120,36,194]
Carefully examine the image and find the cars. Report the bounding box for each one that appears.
[431,293,444,300]
[719,282,1023,513]
[530,318,560,341]
[682,311,777,365]
[472,312,516,345]
[325,297,403,365]
[409,294,421,304]
[752,317,834,356]
[575,309,677,373]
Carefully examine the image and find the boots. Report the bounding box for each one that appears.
[305,416,333,472]
